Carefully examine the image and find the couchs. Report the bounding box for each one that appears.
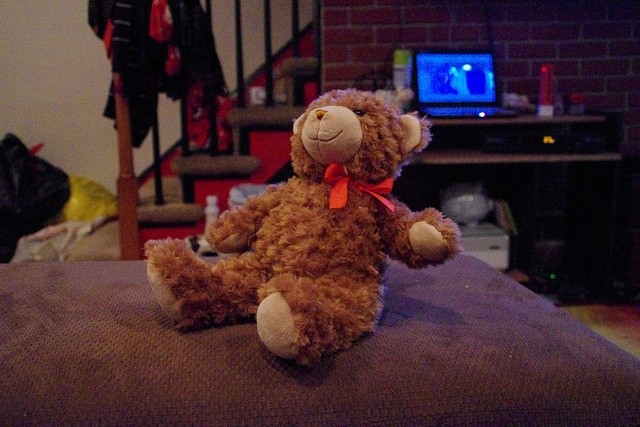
[1,249,638,425]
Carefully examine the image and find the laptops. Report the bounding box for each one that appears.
[411,47,519,119]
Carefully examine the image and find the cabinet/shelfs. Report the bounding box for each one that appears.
[419,115,622,164]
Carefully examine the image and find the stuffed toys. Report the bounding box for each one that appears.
[141,86,464,368]
